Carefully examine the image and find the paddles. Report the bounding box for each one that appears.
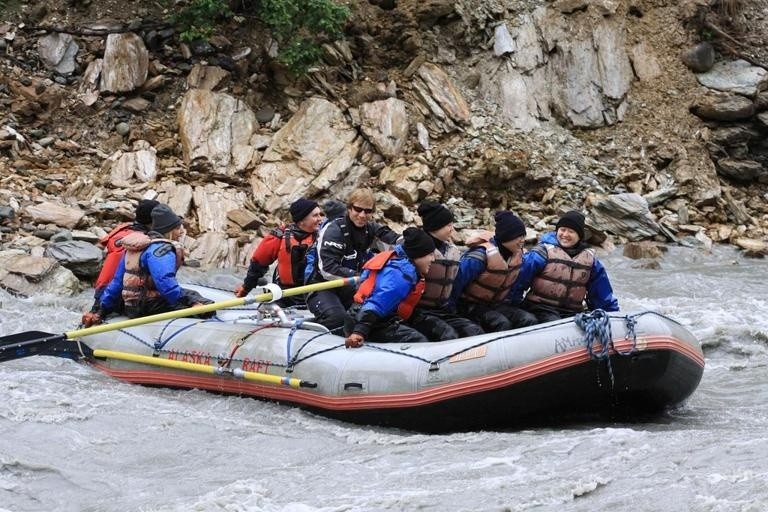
[40,339,318,389]
[0,276,361,363]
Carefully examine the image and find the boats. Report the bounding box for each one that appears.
[66,289,714,437]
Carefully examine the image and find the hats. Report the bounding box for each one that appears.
[402,227,437,260]
[136,199,160,223]
[323,201,346,220]
[416,201,455,232]
[150,203,182,235]
[494,209,527,243]
[555,210,586,237]
[289,198,319,223]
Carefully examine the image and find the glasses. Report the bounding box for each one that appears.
[351,205,375,214]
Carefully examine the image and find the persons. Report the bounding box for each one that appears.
[397,194,489,344]
[448,209,540,333]
[91,202,217,327]
[338,224,437,350]
[233,195,325,319]
[302,187,406,339]
[317,200,349,222]
[81,199,162,324]
[509,209,620,324]
